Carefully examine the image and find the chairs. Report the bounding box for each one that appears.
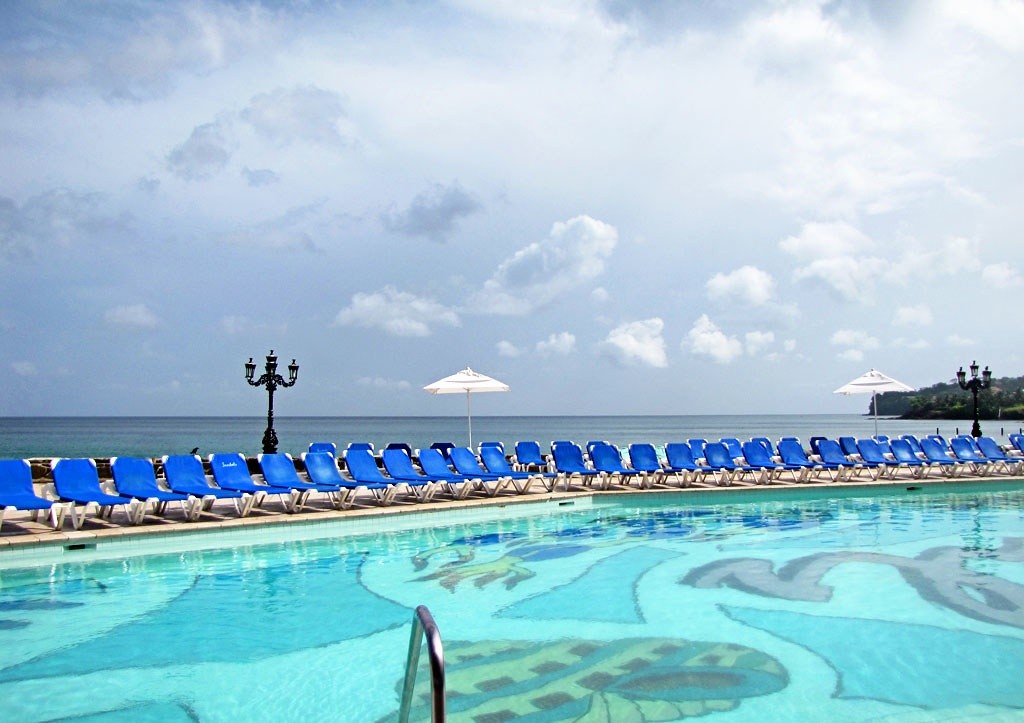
[0,429,1024,536]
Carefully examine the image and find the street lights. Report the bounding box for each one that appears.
[956,360,992,437]
[246,349,299,454]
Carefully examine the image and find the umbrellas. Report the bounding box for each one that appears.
[422,367,511,449]
[832,368,914,440]
